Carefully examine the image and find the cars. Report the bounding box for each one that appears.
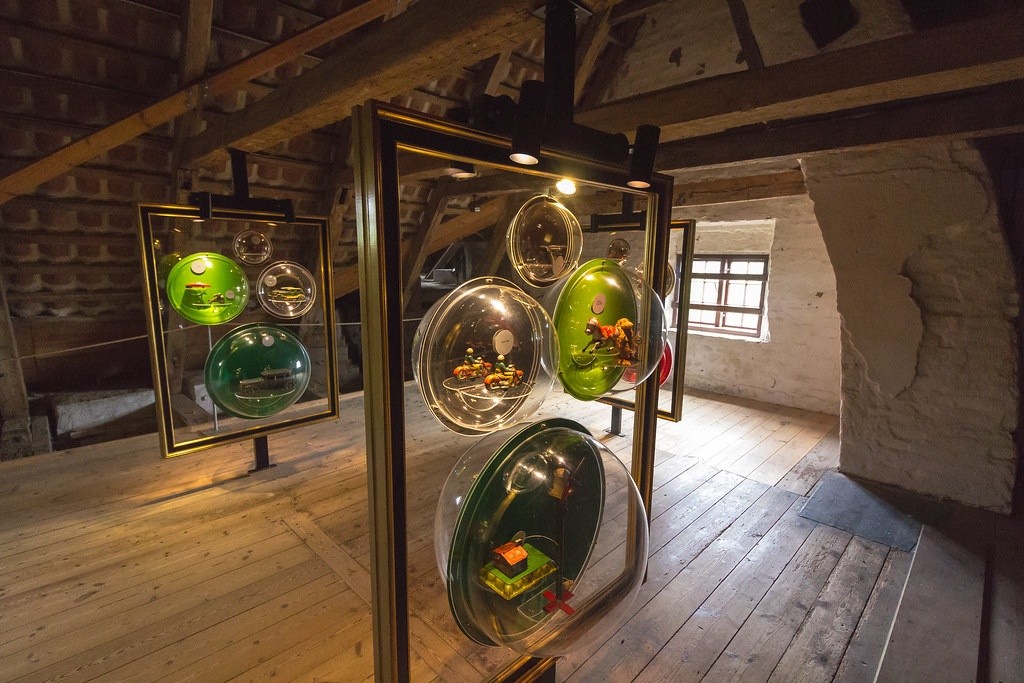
[239,369,295,397]
[267,287,305,301]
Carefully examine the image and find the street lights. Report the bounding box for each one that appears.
[548,467,571,599]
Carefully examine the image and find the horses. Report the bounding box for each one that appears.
[582,316,634,353]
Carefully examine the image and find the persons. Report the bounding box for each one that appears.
[461,346,485,375]
[208,293,228,305]
[494,353,519,390]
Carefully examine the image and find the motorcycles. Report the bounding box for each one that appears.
[453,357,492,383]
[484,365,524,392]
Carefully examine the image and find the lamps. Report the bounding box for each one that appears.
[510,79,548,164]
[451,160,477,178]
[611,124,661,188]
